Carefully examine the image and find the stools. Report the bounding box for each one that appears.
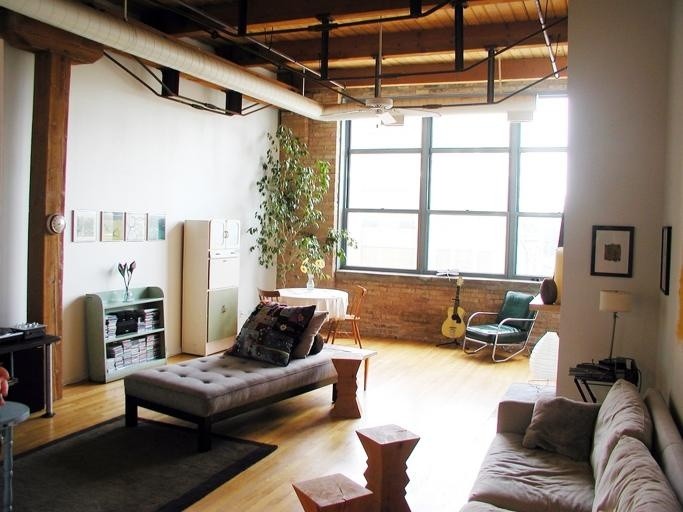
[355,424,420,512]
[290,473,372,512]
[0,400,32,512]
[330,354,362,418]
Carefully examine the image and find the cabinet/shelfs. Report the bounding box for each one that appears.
[85,285,167,385]
[181,218,239,357]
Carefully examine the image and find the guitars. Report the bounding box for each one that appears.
[442,277,466,339]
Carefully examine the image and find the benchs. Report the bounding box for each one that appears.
[124,349,337,452]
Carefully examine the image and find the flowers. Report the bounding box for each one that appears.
[116,259,135,297]
[298,257,332,281]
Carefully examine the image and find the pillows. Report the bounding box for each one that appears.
[520,392,602,464]
[222,300,316,367]
[309,334,323,354]
[292,311,329,359]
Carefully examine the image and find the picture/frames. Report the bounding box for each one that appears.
[71,209,167,242]
[590,225,634,278]
[659,226,670,295]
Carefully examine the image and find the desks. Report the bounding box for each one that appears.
[572,373,614,403]
[325,343,378,392]
[0,326,61,419]
[273,286,349,320]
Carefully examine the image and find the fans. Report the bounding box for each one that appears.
[315,96,441,127]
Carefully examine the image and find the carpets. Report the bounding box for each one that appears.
[0,414,278,512]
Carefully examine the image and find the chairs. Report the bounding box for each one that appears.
[324,284,367,349]
[254,286,280,304]
[462,291,537,364]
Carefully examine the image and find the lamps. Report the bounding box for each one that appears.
[596,289,632,360]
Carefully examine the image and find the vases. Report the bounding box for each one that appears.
[121,291,134,302]
[306,274,314,290]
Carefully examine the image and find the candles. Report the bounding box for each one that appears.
[553,246,562,303]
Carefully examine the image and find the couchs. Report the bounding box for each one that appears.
[454,382,683,511]
